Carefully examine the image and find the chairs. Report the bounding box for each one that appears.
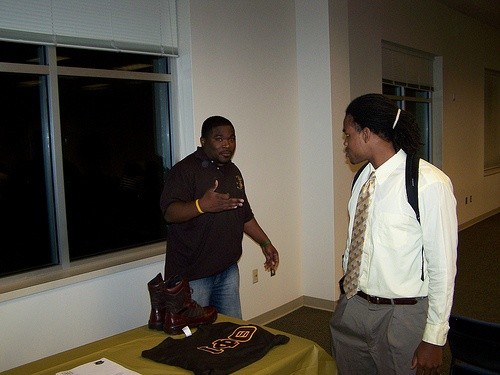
[447,315,500,375]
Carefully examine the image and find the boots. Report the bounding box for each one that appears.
[147,273,217,334]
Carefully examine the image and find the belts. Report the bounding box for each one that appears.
[356,291,418,305]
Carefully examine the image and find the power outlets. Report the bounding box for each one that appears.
[253,269,258,284]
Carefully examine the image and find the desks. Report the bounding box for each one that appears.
[0,312,338,375]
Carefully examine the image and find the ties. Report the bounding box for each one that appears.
[343,171,376,299]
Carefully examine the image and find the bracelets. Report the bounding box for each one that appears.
[195,198,205,214]
[260,239,270,247]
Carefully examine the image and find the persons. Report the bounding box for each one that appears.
[327,93,458,375]
[160,116,279,320]
